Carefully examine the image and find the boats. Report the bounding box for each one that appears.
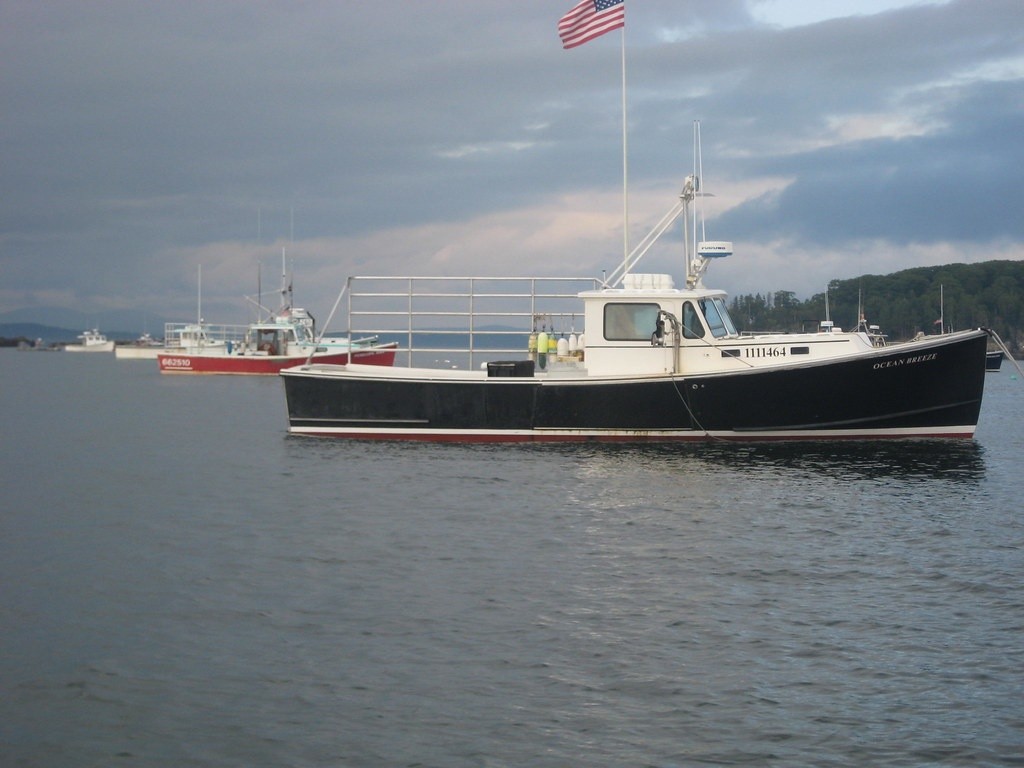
[983,349,1004,373]
[274,0,996,445]
[64,327,115,352]
[158,246,400,375]
[115,322,241,359]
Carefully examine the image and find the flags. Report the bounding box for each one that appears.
[557,0,624,49]
